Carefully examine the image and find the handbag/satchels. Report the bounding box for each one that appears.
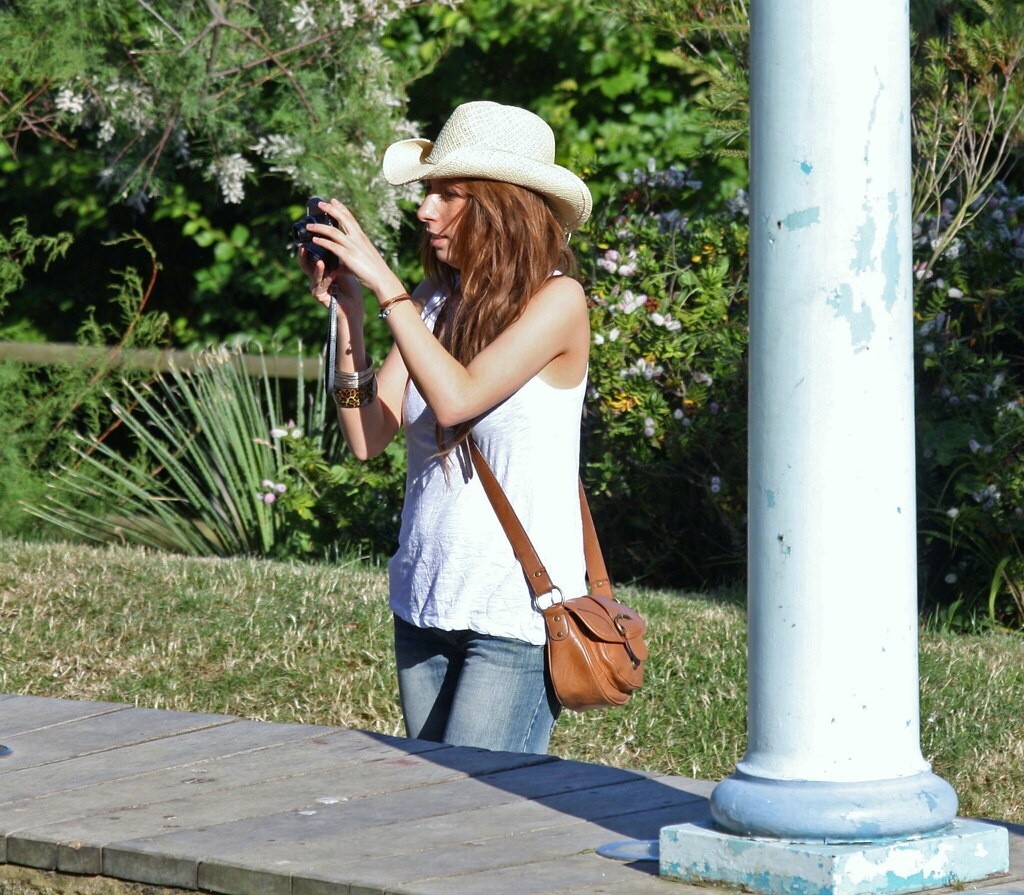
[539,591,649,712]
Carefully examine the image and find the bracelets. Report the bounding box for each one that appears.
[332,351,378,408]
[378,293,415,320]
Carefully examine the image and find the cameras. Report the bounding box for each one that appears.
[292,197,339,271]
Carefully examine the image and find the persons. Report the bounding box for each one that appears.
[297,100,591,755]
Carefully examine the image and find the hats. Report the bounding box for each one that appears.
[382,100,593,235]
